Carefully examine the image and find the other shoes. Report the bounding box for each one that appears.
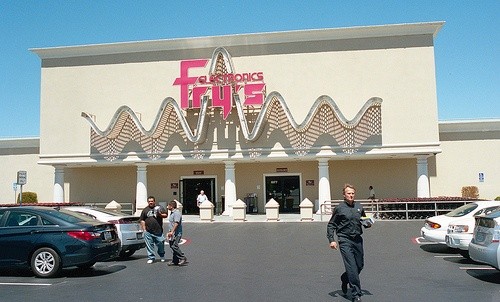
[341,276,347,294]
[354,297,361,302]
[178,260,187,265]
[168,262,175,266]
[147,260,153,263]
[161,258,165,262]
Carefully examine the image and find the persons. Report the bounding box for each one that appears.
[326,183,372,302]
[167,200,187,266]
[140,196,168,264]
[366,185,377,211]
[196,190,207,218]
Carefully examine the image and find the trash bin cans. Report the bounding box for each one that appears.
[158,201,168,215]
[245,193,258,213]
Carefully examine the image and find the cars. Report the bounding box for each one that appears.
[0,205,122,279]
[468,206,500,273]
[444,202,500,261]
[48,205,147,261]
[420,200,500,245]
